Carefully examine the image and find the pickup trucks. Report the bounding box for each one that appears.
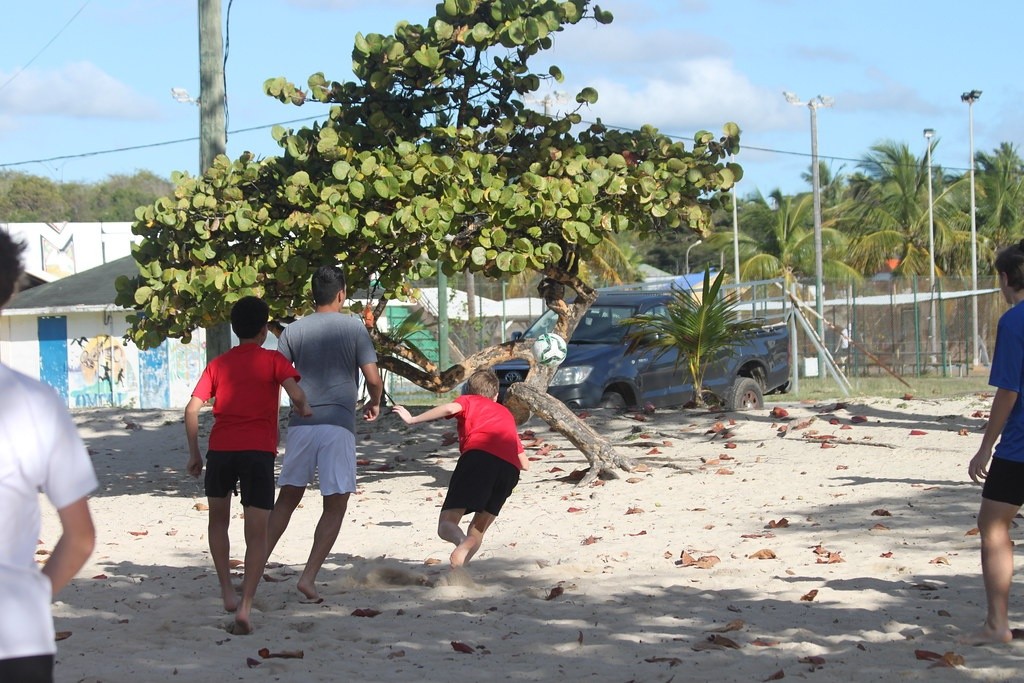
[462,289,792,411]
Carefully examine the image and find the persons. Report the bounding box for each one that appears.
[394,369,525,571]
[237,264,383,606]
[954,240,1024,650]
[0,227,100,683]
[177,295,314,635]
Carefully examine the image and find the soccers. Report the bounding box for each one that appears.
[532,333,567,366]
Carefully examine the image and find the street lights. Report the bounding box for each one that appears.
[924,128,940,371]
[526,89,570,142]
[781,91,833,381]
[962,90,982,376]
[685,240,701,273]
